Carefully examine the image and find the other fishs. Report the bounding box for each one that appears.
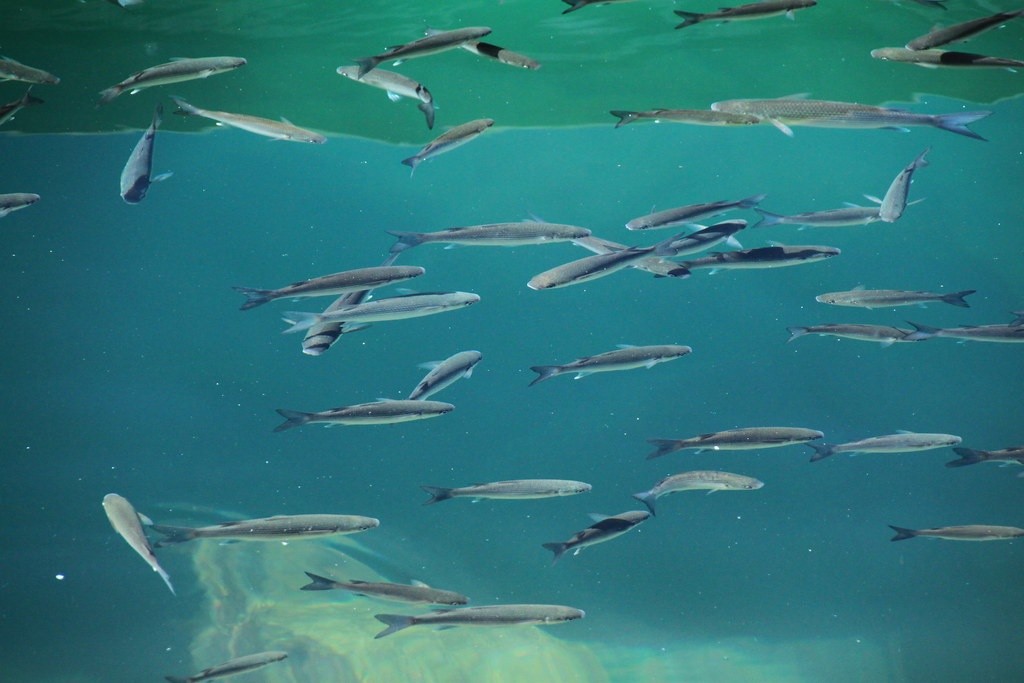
[0,0,1024,682]
